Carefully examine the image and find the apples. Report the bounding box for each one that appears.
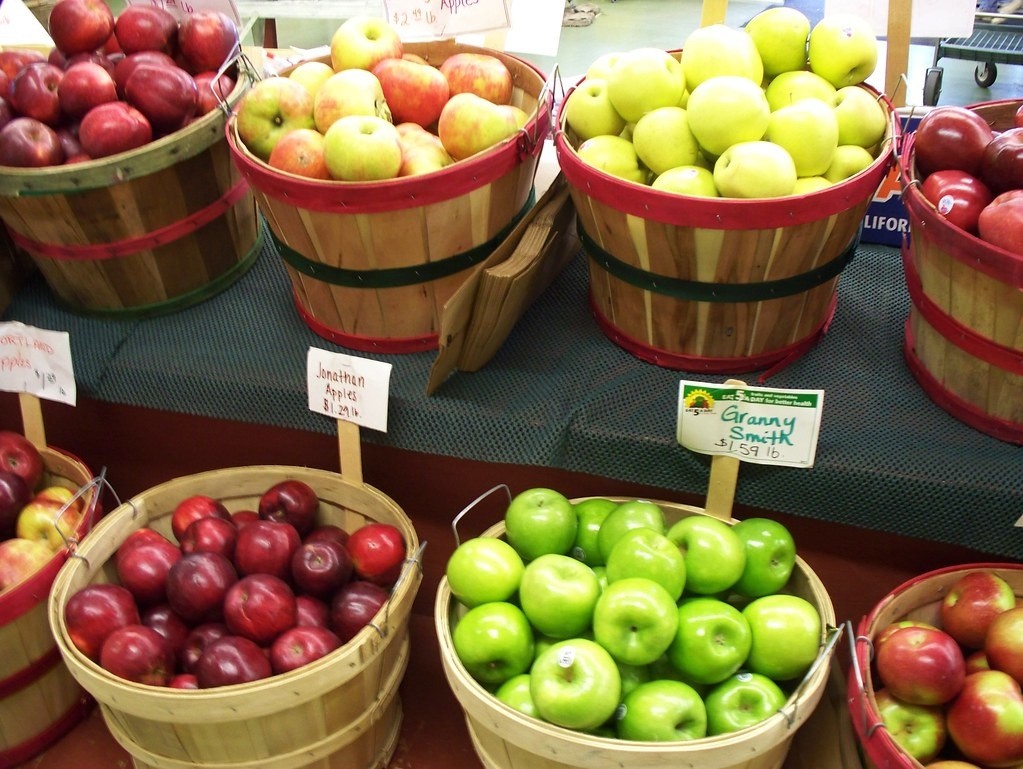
[446,487,821,741]
[65,481,406,689]
[0,431,86,591]
[915,104,1023,256]
[0,0,239,168]
[566,6,885,199]
[239,14,529,181]
[873,572,1023,769]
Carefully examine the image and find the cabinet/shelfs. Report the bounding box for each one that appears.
[1,217,1023,769]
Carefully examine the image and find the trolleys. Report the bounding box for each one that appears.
[921,11,1023,106]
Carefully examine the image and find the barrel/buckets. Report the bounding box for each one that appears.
[848,561,1023,769]
[48,466,422,768]
[224,41,555,352]
[0,449,103,769]
[558,49,898,371]
[902,97,1023,444]
[0,53,264,318]
[432,485,843,769]
